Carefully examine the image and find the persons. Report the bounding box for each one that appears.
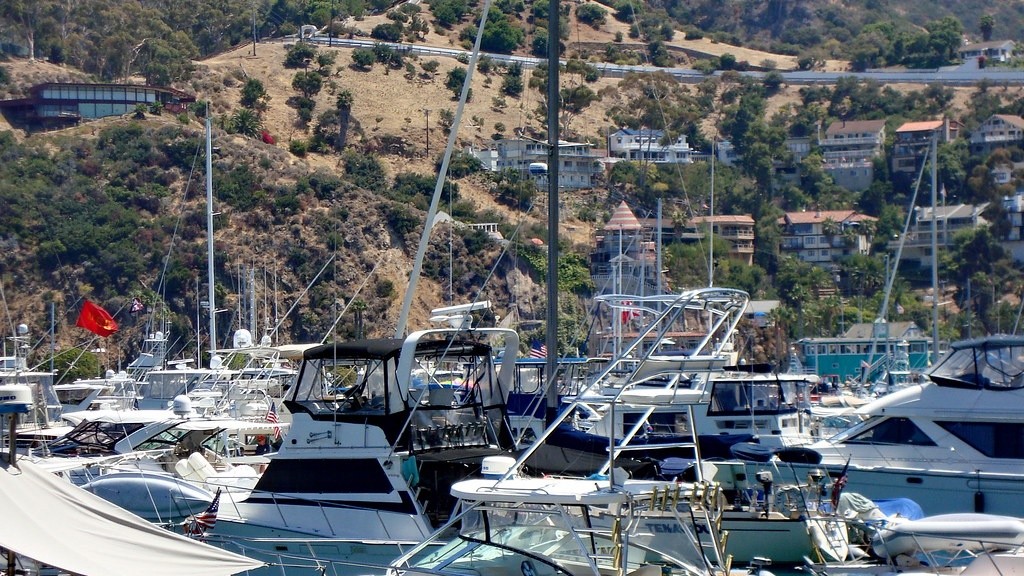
[255,434,270,455]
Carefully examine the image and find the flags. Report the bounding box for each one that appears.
[621,301,641,324]
[183,492,219,535]
[76,299,117,337]
[268,404,283,438]
[530,342,547,359]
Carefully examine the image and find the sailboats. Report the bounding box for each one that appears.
[1,0,1023,576]
